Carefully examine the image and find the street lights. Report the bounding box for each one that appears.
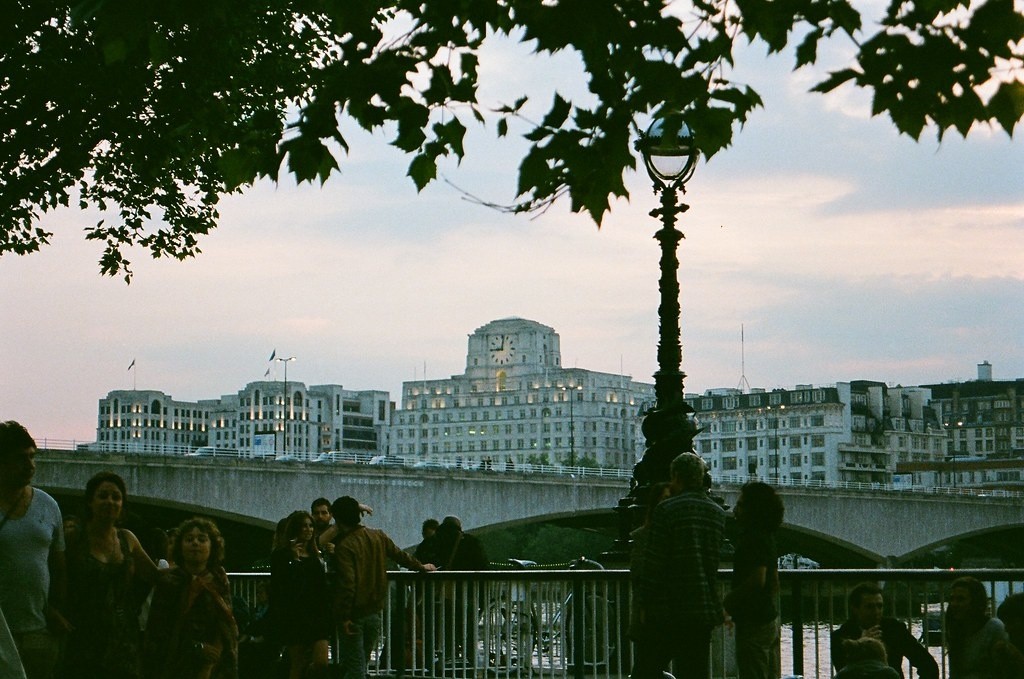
[275,357,297,455]
[601,110,735,558]
[766,405,785,484]
[562,386,583,474]
[944,422,963,495]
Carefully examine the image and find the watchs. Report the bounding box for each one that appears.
[194,641,204,659]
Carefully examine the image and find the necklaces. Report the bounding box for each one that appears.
[94,531,115,564]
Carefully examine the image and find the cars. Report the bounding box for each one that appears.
[369,455,411,466]
[312,452,354,462]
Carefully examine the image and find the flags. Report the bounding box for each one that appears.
[128,360,134,370]
[265,368,269,376]
[269,349,275,361]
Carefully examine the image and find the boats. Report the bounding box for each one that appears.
[920,597,998,647]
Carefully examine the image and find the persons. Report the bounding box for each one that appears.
[831,579,1024,679]
[365,448,372,461]
[49,473,505,679]
[456,457,532,471]
[626,450,783,679]
[0,421,67,679]
[318,450,328,459]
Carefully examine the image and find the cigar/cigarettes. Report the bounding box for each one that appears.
[290,539,296,541]
[436,565,442,570]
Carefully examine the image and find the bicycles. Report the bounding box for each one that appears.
[466,555,624,677]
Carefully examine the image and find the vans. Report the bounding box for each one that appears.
[185,446,238,456]
